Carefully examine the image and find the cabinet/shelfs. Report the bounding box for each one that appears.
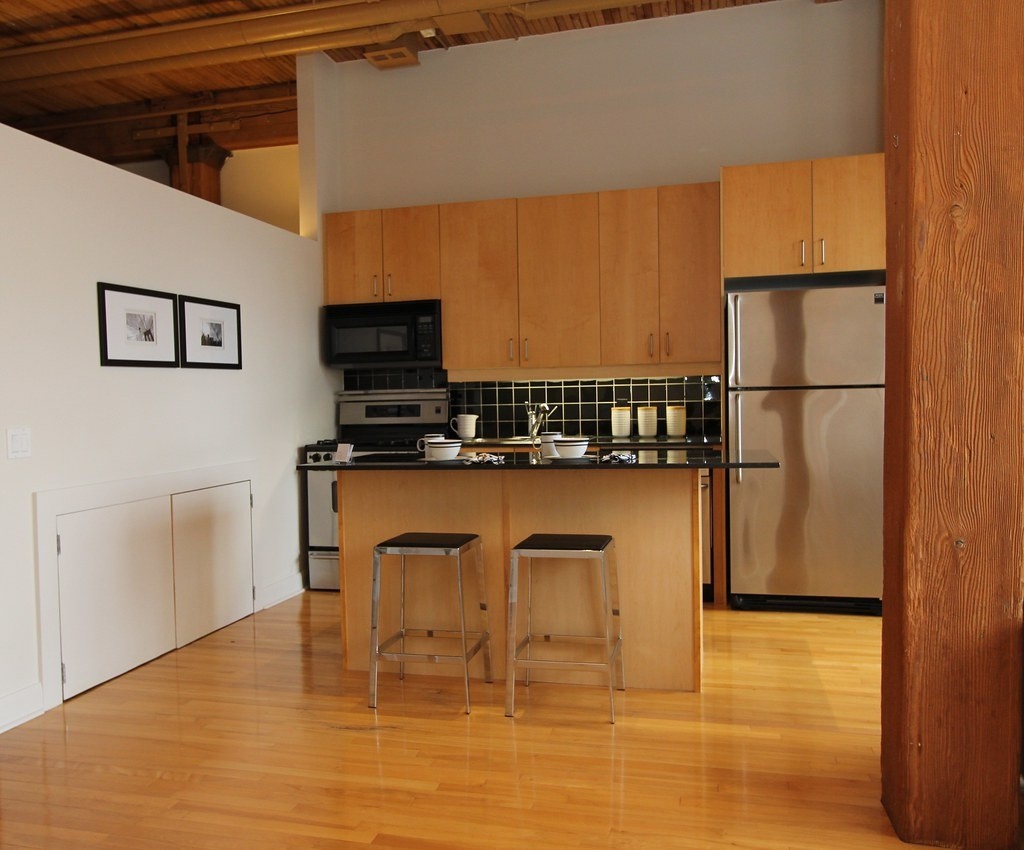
[320,181,726,383]
[460,445,716,603]
[719,153,886,299]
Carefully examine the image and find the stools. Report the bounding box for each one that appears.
[367,532,494,714]
[504,533,626,723]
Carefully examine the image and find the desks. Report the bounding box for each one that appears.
[296,450,782,693]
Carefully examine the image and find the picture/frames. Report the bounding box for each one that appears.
[178,294,244,369]
[98,283,180,369]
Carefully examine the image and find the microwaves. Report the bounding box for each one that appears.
[322,301,442,369]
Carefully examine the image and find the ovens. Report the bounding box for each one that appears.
[306,470,341,590]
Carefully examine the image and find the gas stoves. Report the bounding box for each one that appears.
[305,425,447,451]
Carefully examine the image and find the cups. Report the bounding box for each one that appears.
[450,414,479,439]
[639,438,658,464]
[611,438,631,456]
[416,433,445,459]
[666,405,686,437]
[637,407,657,437]
[666,439,687,464]
[611,407,630,437]
[533,431,562,457]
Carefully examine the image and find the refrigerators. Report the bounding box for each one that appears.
[725,285,886,617]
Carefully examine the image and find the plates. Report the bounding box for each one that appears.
[544,455,600,459]
[417,458,468,461]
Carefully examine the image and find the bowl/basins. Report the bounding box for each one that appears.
[428,439,462,459]
[553,437,589,458]
